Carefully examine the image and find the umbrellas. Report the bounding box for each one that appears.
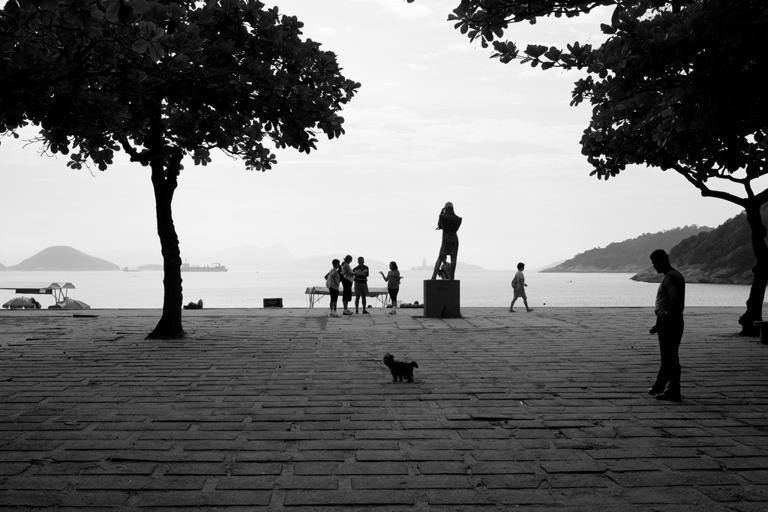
[1,295,42,309]
[61,297,92,312]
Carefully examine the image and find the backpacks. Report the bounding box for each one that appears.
[512,276,518,288]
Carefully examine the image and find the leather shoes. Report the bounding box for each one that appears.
[647,387,664,395]
[655,392,682,402]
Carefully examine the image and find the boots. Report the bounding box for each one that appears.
[510,308,516,312]
[526,308,533,311]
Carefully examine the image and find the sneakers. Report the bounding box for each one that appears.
[330,308,397,317]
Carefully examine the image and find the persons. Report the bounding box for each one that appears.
[438,256,451,281]
[340,255,358,316]
[508,263,534,313]
[430,202,462,281]
[353,257,371,315]
[324,258,342,318]
[648,248,685,402]
[379,261,404,315]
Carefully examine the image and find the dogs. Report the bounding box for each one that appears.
[382,354,419,384]
